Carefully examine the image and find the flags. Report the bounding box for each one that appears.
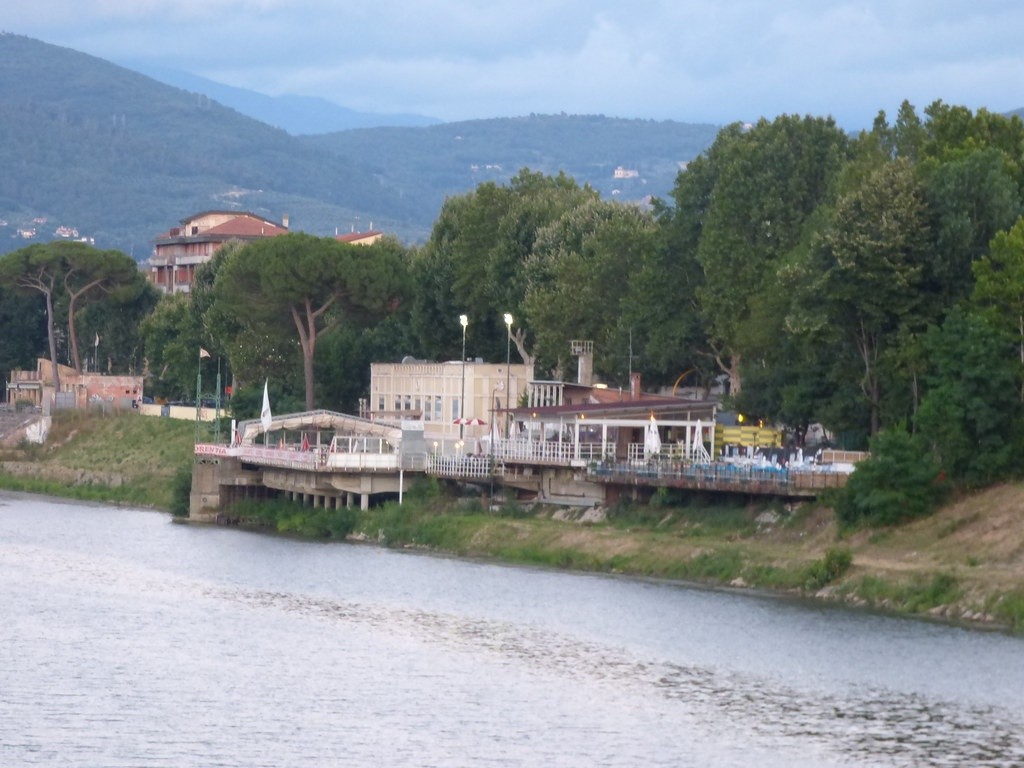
[199,347,210,358]
[260,381,272,432]
[302,433,310,450]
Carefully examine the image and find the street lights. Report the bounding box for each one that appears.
[460,314,468,458]
[502,312,513,452]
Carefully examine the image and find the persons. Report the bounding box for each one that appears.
[795,443,803,463]
[817,437,830,459]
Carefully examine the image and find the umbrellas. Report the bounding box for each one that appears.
[693,418,702,452]
[235,429,241,448]
[453,417,487,425]
[645,414,661,460]
